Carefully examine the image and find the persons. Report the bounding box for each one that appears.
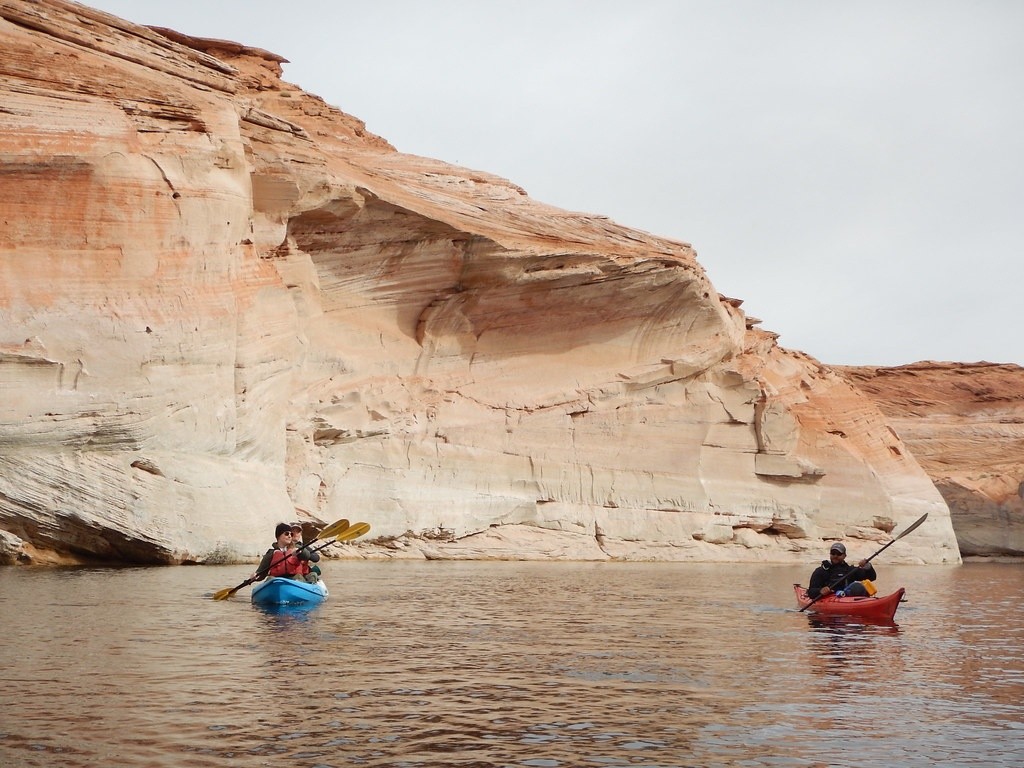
[250,522,320,584]
[808,543,876,598]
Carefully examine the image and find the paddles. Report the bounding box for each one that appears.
[210,519,351,598]
[796,512,930,614]
[214,521,371,594]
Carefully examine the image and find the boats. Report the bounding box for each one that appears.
[793,583,908,626]
[252,565,329,605]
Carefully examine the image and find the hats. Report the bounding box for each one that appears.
[275,523,292,538]
[289,522,303,531]
[830,542,846,554]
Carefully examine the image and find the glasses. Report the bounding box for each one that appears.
[284,531,294,537]
[830,551,845,556]
